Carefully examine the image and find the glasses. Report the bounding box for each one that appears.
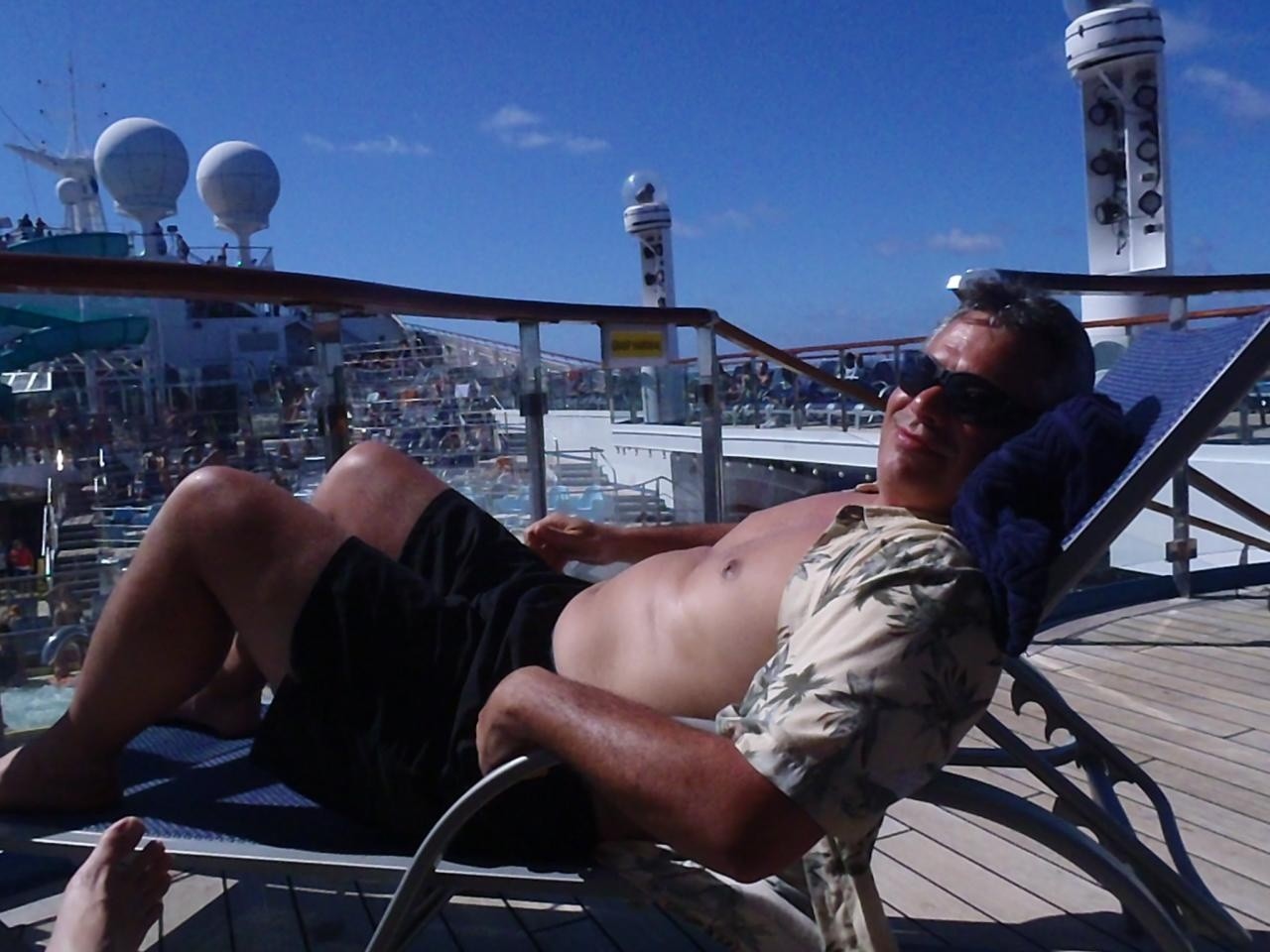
[897,349,1030,436]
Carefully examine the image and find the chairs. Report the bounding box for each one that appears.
[685,358,899,430]
[21,311,1268,952]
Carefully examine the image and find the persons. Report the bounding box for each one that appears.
[0,274,1098,889]
[0,209,776,684]
[38,816,176,952]
